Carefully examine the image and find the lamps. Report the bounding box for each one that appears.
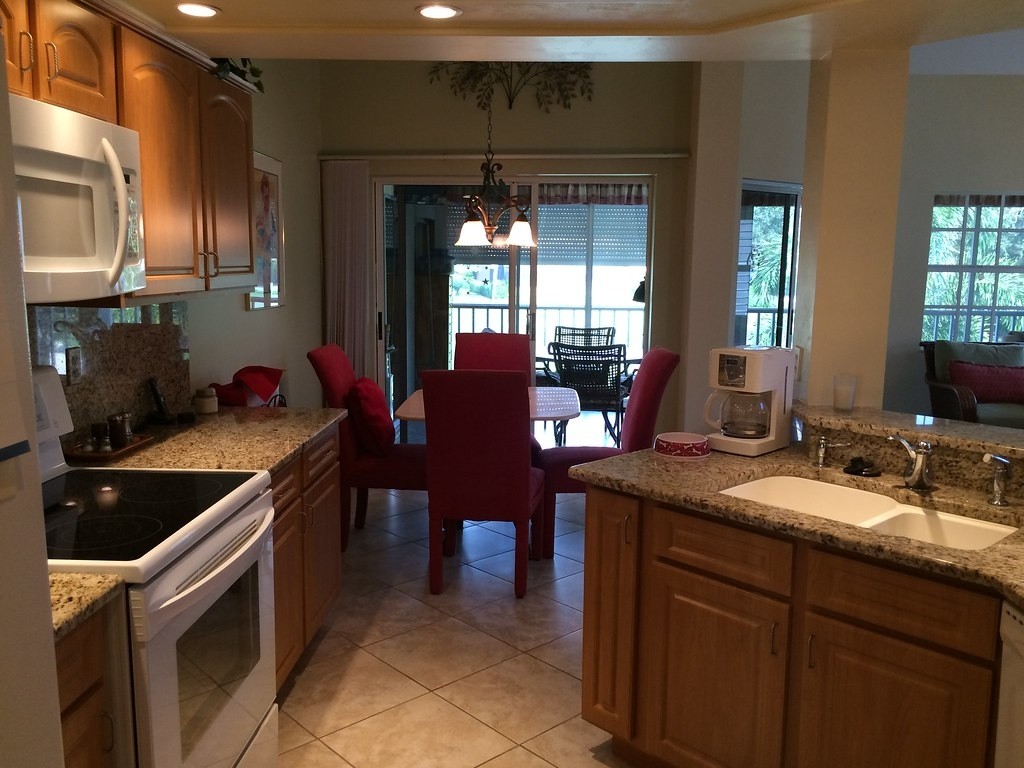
[632,275,646,302]
[454,67,537,248]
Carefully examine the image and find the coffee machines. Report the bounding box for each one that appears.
[709,342,796,457]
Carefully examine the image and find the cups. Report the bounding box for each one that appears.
[90,422,108,452]
[833,374,858,411]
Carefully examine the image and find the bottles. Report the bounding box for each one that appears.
[194,387,218,414]
[106,412,133,450]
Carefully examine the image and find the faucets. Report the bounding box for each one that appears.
[982,453,1013,507]
[812,436,852,468]
[907,451,919,452]
[886,434,936,491]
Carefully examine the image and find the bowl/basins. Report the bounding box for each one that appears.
[654,433,710,458]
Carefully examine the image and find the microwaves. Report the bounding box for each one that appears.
[7,93,147,302]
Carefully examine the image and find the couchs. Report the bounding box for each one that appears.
[918,340,1024,430]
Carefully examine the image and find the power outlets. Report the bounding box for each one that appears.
[66,346,82,385]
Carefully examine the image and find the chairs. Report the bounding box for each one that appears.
[306,326,681,601]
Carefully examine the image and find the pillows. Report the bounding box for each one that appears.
[948,360,1024,405]
[345,377,396,459]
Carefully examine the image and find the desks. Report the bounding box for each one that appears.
[393,382,581,427]
[535,351,644,444]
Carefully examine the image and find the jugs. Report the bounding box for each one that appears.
[703,390,771,439]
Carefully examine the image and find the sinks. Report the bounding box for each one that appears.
[864,504,1019,550]
[717,475,903,528]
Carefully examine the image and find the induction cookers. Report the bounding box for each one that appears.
[31,365,272,585]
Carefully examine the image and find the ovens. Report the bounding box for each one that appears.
[128,485,280,768]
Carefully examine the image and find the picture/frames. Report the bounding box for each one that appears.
[245,149,285,312]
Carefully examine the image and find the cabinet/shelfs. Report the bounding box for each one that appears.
[0,0,257,309]
[581,482,1004,768]
[55,605,115,768]
[268,421,345,695]
